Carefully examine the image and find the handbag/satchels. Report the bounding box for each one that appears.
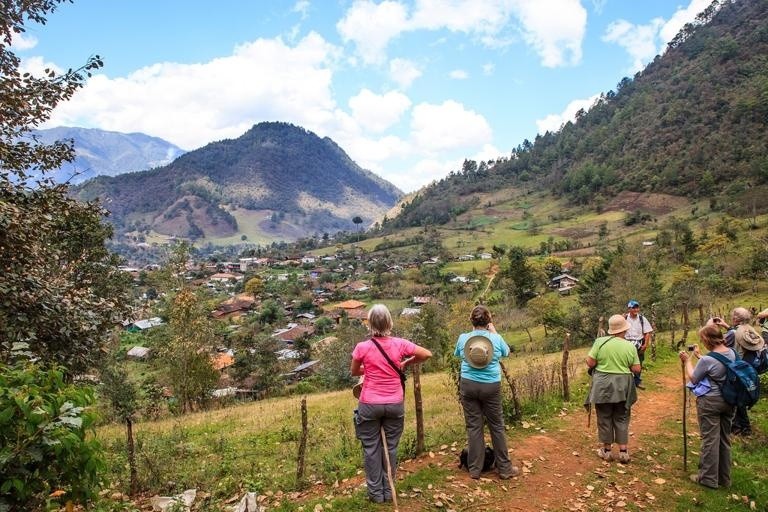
[401,372,408,391]
[462,446,496,472]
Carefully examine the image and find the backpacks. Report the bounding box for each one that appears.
[706,348,768,407]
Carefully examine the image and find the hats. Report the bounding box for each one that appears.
[627,301,638,308]
[465,336,494,369]
[608,315,630,334]
[735,324,765,350]
[353,375,364,398]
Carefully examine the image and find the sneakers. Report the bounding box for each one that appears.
[500,467,518,479]
[619,451,630,463]
[636,384,646,390]
[689,474,698,482]
[598,447,612,461]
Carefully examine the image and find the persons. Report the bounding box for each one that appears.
[350,304,432,504]
[623,301,654,389]
[678,325,735,489]
[756,308,768,370]
[586,314,641,463]
[715,308,756,439]
[453,305,520,479]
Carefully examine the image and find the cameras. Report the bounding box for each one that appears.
[687,345,695,351]
[713,318,720,323]
